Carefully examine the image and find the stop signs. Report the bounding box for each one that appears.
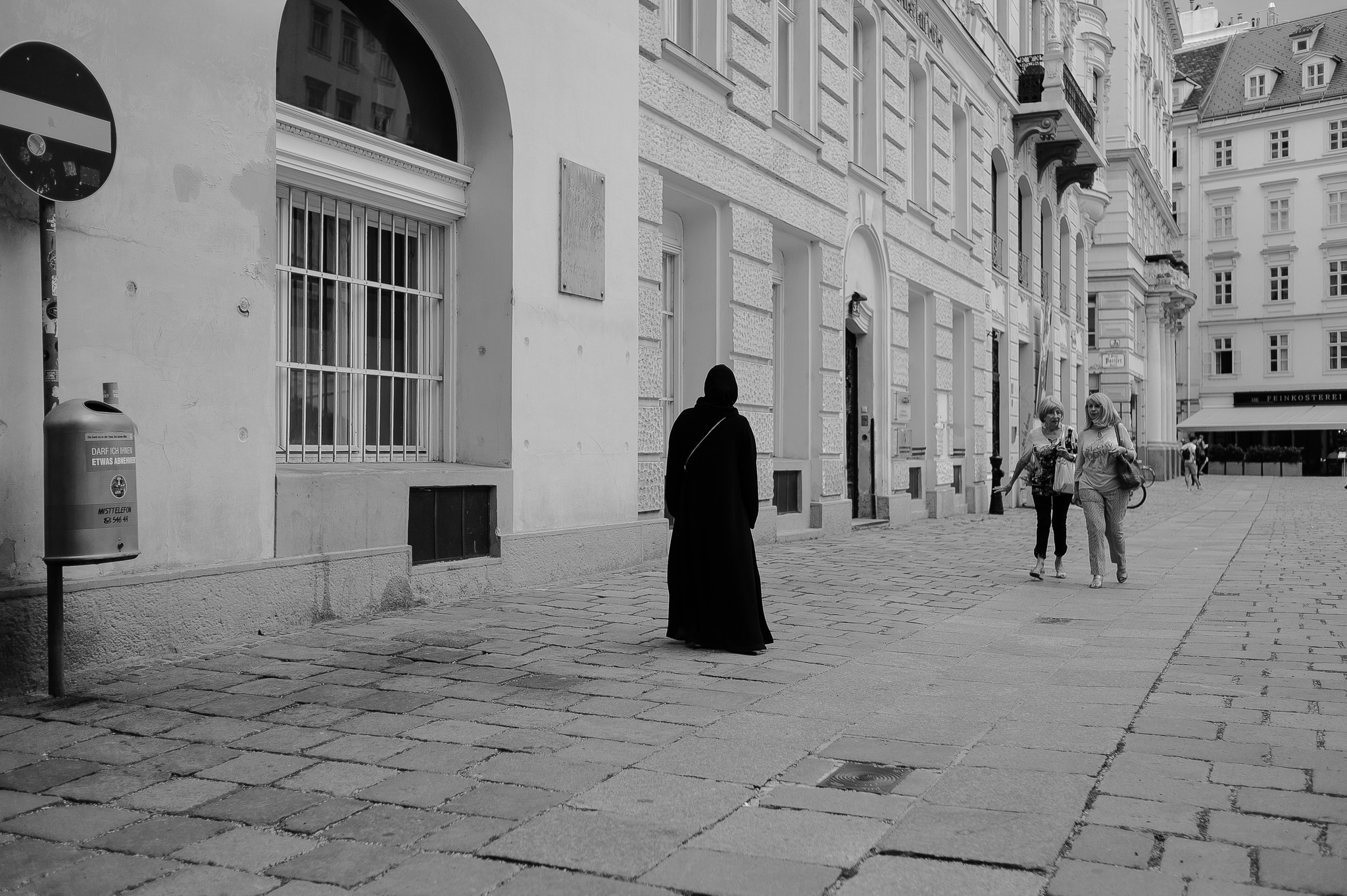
[0,40,118,205]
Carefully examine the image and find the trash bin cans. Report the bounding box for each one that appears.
[42,398,141,566]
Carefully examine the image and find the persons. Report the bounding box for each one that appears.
[1074,393,1137,589]
[992,396,1080,581]
[1178,435,1209,492]
[664,364,774,656]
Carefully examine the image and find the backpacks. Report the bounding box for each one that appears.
[1182,448,1190,460]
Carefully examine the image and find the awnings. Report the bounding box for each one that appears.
[1175,406,1347,433]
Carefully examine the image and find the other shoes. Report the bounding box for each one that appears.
[1187,488,1192,492]
[1197,486,1203,490]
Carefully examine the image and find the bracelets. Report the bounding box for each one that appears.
[1124,447,1128,456]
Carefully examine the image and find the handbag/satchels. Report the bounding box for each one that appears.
[664,470,685,518]
[1115,453,1146,491]
[1053,424,1076,494]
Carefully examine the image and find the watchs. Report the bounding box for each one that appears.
[1072,454,1076,462]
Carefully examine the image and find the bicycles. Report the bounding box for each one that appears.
[1126,484,1147,509]
[1137,459,1156,488]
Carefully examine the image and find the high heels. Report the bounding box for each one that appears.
[1029,567,1045,580]
[1055,564,1067,579]
[1116,562,1128,583]
[1089,575,1104,589]
